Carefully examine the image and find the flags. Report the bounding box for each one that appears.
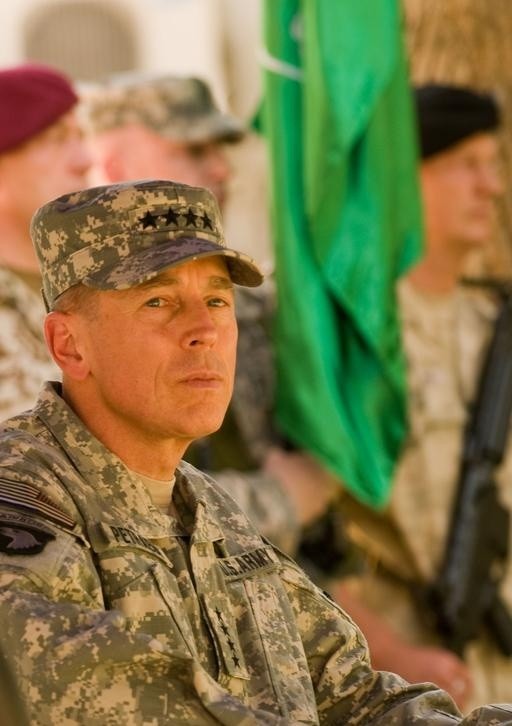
[248,0,428,519]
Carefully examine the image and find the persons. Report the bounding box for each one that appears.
[390,82,512,710]
[79,69,475,714]
[0,63,93,421]
[0,178,512,726]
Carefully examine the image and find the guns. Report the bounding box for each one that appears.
[430,279,511,655]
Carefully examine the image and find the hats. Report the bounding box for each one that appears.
[414,86,502,156]
[0,67,79,153]
[30,180,263,316]
[88,76,244,147]
